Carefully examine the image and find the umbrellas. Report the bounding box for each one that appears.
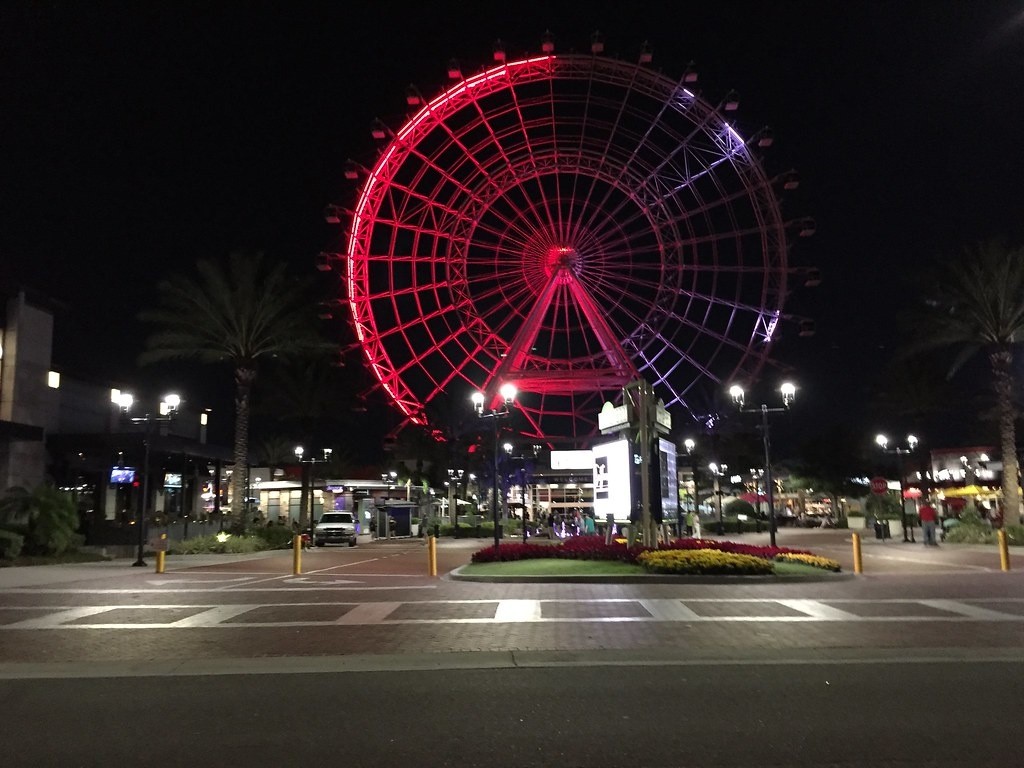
[944,484,994,497]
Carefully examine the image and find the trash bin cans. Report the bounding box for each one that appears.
[494,525,504,538]
[874,518,890,538]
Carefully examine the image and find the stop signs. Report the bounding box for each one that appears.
[871,478,888,496]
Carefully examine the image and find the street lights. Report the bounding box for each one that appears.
[381,472,397,500]
[729,382,795,546]
[295,447,333,547]
[750,468,764,532]
[710,463,728,536]
[876,434,918,542]
[504,443,542,544]
[448,469,464,539]
[118,393,180,567]
[676,439,695,539]
[472,384,515,547]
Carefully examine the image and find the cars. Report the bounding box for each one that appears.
[219,497,260,515]
[201,495,223,512]
[313,512,360,547]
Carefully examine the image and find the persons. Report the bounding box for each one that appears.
[525,508,564,540]
[573,510,595,534]
[253,506,299,532]
[919,501,938,544]
[685,510,693,536]
[692,511,701,539]
[370,517,377,540]
